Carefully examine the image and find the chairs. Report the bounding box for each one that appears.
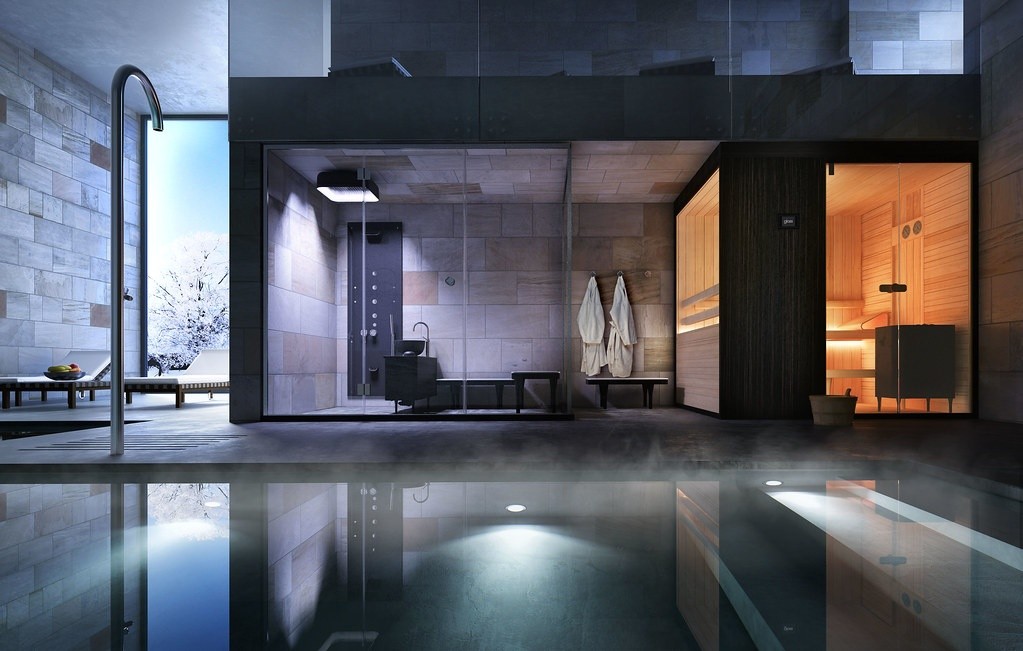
[124,349,230,408]
[0,350,114,409]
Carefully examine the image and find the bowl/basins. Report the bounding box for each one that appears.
[808,395,858,424]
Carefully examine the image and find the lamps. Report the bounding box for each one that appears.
[316,171,379,203]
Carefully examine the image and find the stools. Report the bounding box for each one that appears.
[511,371,560,413]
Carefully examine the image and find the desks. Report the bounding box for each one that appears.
[16,381,112,408]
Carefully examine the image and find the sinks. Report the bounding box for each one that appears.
[394,340,426,356]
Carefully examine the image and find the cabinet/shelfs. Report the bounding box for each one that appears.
[383,355,438,414]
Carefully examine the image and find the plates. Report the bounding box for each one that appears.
[43,371,86,380]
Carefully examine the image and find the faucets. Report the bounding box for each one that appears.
[412,321,430,357]
[111,63,165,457]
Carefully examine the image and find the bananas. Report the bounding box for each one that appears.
[47,364,72,372]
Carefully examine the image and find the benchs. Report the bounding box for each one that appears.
[435,378,515,410]
[586,377,669,409]
